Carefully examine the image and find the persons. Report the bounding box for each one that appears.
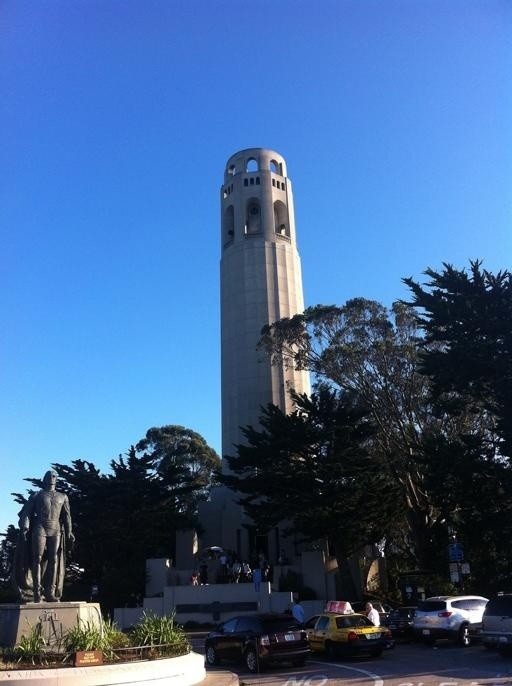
[191,545,271,586]
[291,600,304,624]
[18,470,76,602]
[365,602,381,626]
[278,548,289,564]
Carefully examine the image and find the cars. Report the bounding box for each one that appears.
[204,613,312,673]
[350,600,418,640]
[409,596,489,648]
[479,592,512,650]
[302,601,392,658]
